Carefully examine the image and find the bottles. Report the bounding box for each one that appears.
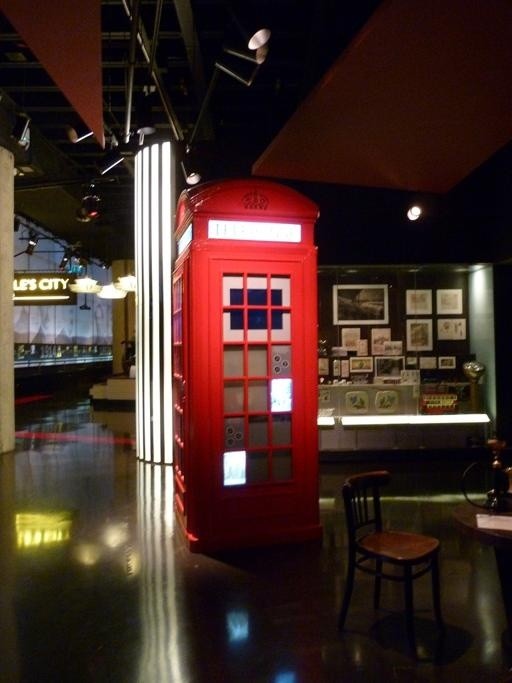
[462,352,487,384]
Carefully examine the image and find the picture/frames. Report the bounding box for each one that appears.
[341,327,392,355]
[332,283,390,325]
[439,355,457,369]
[349,355,406,380]
[405,288,467,351]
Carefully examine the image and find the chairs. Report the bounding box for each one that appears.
[339,469,443,653]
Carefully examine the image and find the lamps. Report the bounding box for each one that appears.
[26,235,71,267]
[66,121,123,175]
[212,11,271,88]
[9,111,30,141]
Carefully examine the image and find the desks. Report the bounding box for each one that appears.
[451,497,512,650]
